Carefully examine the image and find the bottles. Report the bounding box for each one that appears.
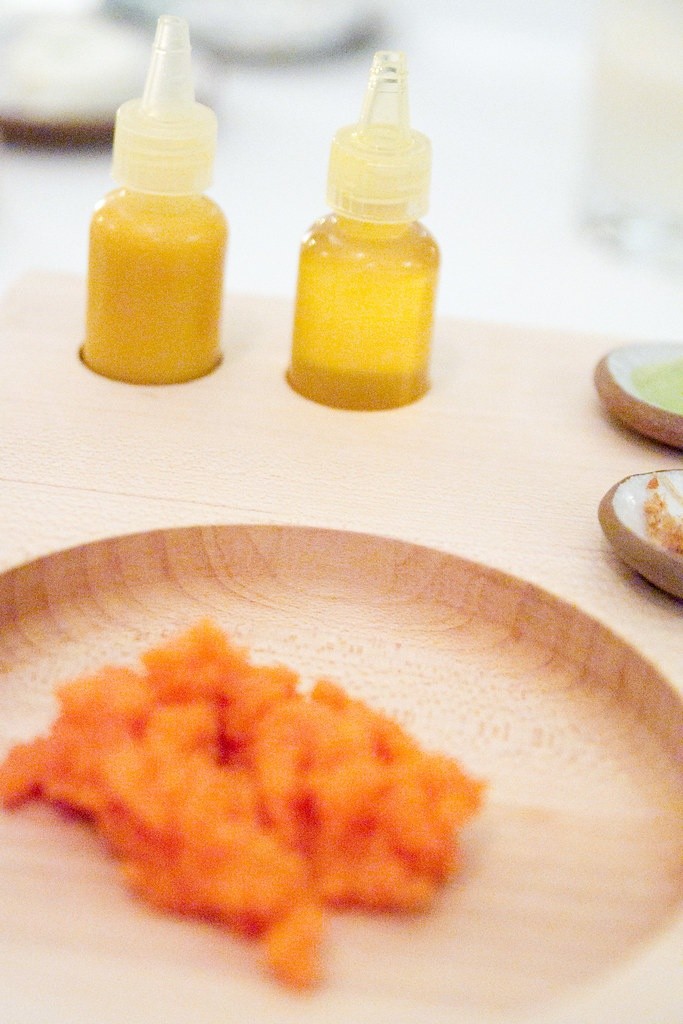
[288,50,443,411]
[81,15,230,384]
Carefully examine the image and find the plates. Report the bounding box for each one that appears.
[598,469,683,599]
[0,524,683,1024]
[595,340,683,452]
